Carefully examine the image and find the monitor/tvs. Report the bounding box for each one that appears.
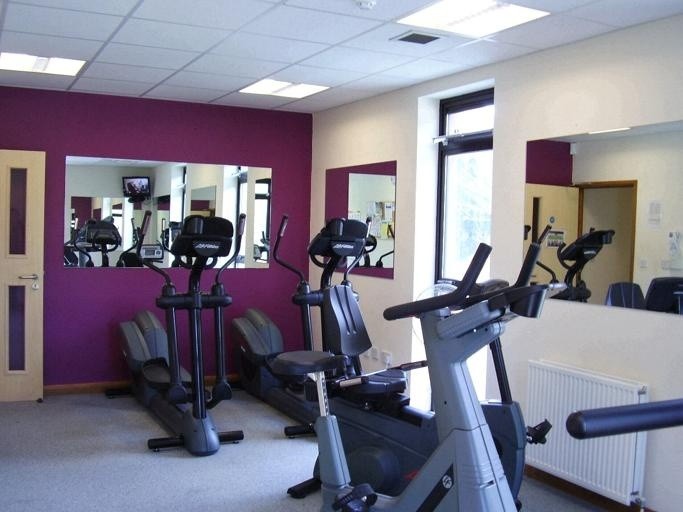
[122,176,150,204]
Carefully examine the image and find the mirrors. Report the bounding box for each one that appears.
[523,121,683,316]
[63,155,273,269]
[325,159,397,280]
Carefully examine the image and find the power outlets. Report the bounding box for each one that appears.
[382,352,393,365]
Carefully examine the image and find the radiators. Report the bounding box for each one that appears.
[522,357,649,512]
